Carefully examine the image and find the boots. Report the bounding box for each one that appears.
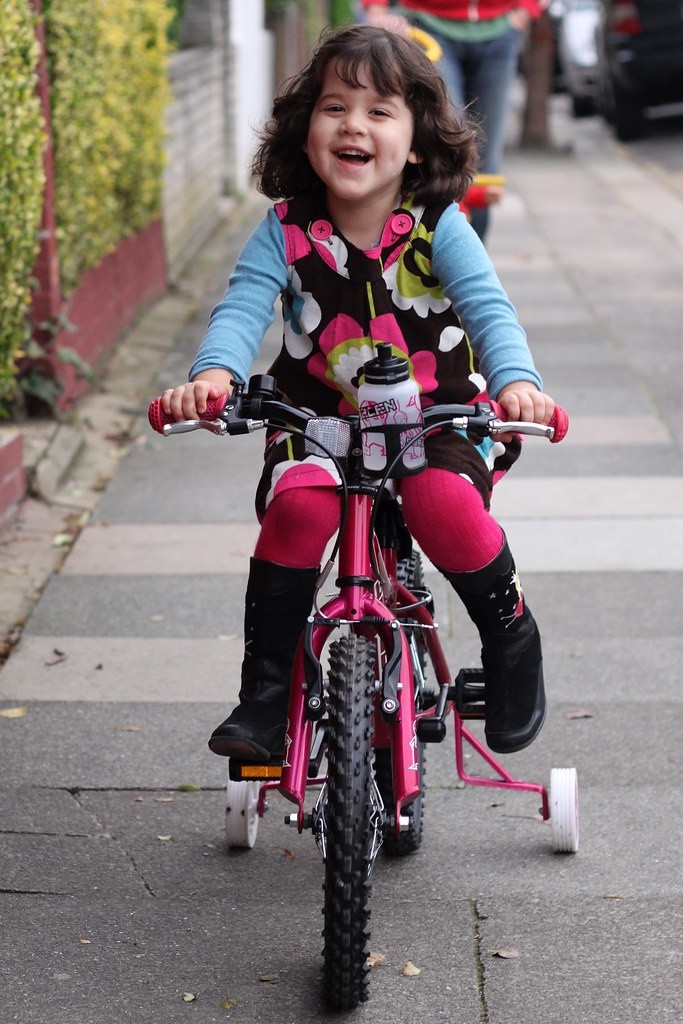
[428,523,548,754]
[208,556,321,759]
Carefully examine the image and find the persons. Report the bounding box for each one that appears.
[361,0,552,244]
[162,26,554,758]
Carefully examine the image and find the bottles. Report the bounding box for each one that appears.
[351,342,429,479]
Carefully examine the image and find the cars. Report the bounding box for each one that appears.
[558,0,683,143]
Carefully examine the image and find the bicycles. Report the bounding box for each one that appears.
[131,380,585,1012]
